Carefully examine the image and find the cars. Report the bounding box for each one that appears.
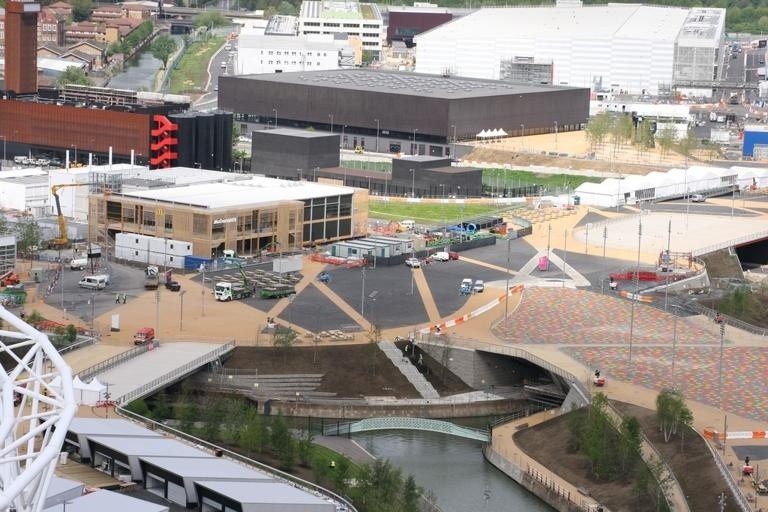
[692,194,707,202]
[224,44,232,50]
[733,46,742,59]
[729,93,739,104]
[220,61,226,69]
[14,155,64,166]
[405,257,420,268]
[214,85,219,91]
[760,60,764,63]
[709,112,725,124]
[448,252,458,260]
[461,277,484,293]
[134,327,154,345]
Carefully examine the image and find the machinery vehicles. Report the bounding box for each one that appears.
[222,242,279,264]
[47,181,103,249]
[259,285,296,298]
[214,261,254,303]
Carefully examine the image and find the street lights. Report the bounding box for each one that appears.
[88,292,95,329]
[313,166,320,183]
[58,259,63,307]
[413,128,418,155]
[451,125,457,158]
[272,109,278,128]
[365,175,371,189]
[520,124,524,137]
[374,119,379,152]
[554,121,558,147]
[409,169,415,198]
[328,113,333,133]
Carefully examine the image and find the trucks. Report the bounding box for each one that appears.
[144,266,160,290]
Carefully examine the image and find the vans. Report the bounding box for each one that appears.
[431,252,449,262]
[399,219,416,228]
[70,259,88,271]
[78,276,106,290]
